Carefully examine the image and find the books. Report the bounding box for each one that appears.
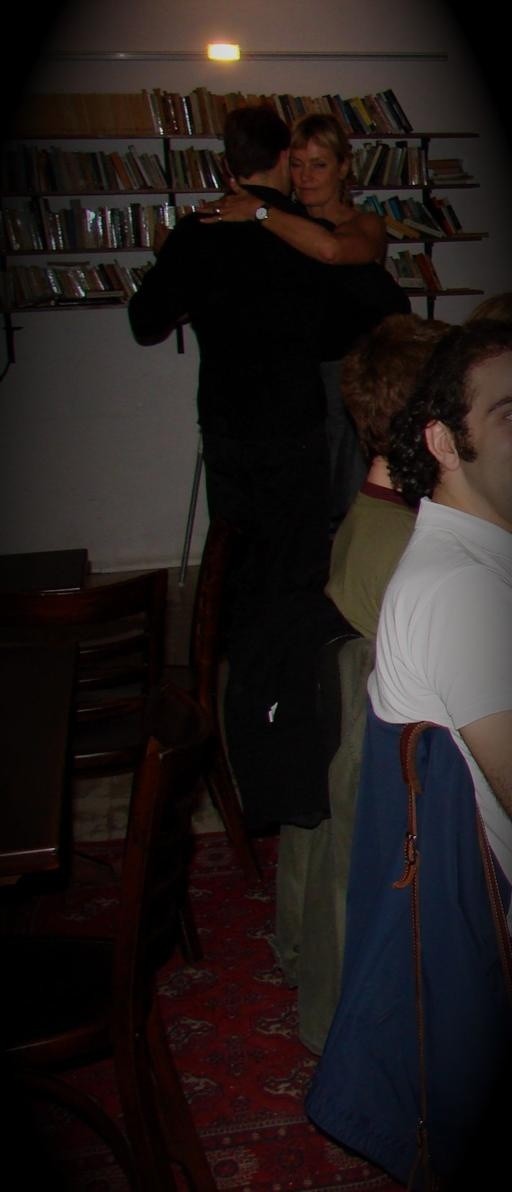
[1,259,154,300]
[386,250,443,293]
[169,140,478,191]
[1,88,412,139]
[356,193,462,239]
[2,145,169,196]
[3,199,208,252]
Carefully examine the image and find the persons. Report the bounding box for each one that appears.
[326,294,512,879]
[194,113,390,531]
[125,102,412,835]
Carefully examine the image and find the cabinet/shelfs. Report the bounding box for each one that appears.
[0,132,490,365]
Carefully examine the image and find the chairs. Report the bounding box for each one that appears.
[302,688,512,1192]
[271,601,377,1058]
